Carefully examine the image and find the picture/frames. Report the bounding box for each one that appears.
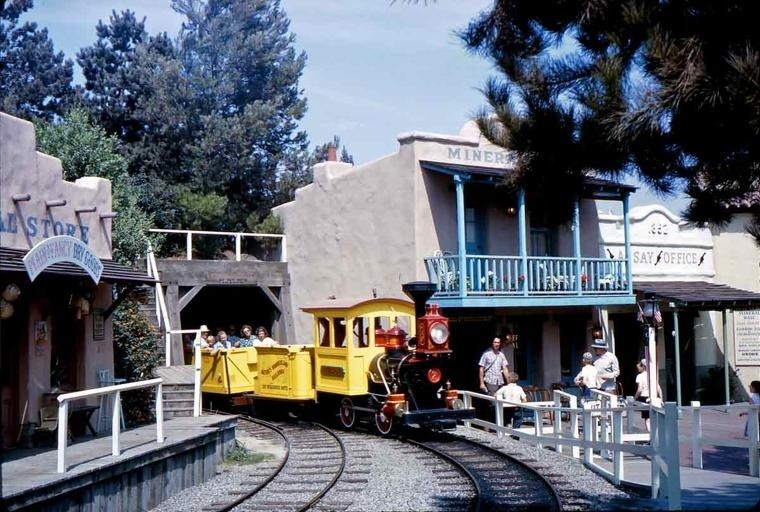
[92,305,106,340]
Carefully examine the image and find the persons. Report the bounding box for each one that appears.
[492,371,527,442]
[739,380,760,441]
[590,338,621,395]
[478,335,511,396]
[633,358,664,434]
[573,352,602,397]
[182,320,281,353]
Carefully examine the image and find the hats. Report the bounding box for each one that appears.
[198,324,209,332]
[591,338,609,349]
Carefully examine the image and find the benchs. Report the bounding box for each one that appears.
[519,381,625,425]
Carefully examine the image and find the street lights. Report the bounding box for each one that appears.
[637,290,663,406]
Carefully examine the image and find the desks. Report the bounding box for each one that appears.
[66,406,100,441]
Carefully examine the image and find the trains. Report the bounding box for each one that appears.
[187,282,475,445]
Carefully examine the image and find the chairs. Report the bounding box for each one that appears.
[431,249,626,292]
[34,404,58,447]
